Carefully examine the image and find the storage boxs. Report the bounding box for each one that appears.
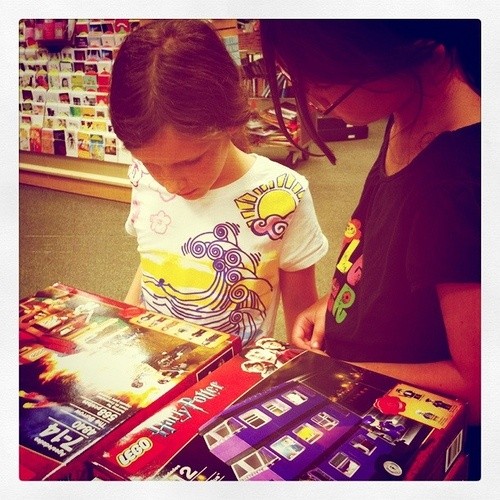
[19,278,471,480]
[317,118,371,142]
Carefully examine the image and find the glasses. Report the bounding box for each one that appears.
[304,77,366,117]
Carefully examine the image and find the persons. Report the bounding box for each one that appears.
[258,18,481,423]
[108,19,330,351]
